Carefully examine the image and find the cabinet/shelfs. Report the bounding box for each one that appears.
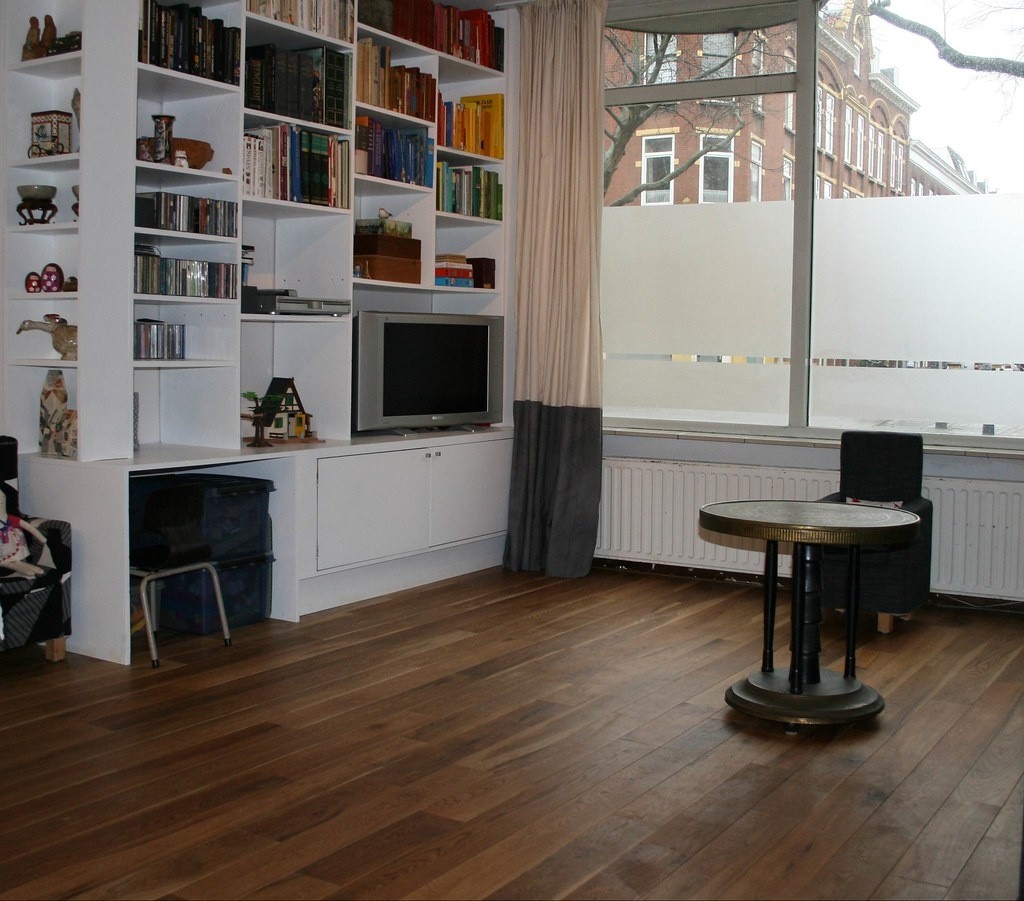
[0,0,520,664]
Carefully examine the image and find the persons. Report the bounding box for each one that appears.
[0,489,49,577]
[24,14,56,55]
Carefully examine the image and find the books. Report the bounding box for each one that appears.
[246,44,352,130]
[243,123,349,210]
[358,0,504,72]
[356,37,435,123]
[436,161,503,221]
[354,116,434,187]
[246,0,354,44]
[437,89,505,160]
[435,254,474,288]
[133,243,236,299]
[135,191,238,238]
[137,0,241,86]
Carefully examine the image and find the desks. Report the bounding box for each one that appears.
[699,498,922,727]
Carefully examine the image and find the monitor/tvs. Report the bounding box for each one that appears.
[354,311,504,437]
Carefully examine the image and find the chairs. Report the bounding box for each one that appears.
[0,435,74,662]
[809,429,933,633]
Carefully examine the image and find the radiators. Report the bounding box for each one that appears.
[592,459,1024,605]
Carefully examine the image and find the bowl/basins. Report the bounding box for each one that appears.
[17,186,57,199]
[72,184,79,200]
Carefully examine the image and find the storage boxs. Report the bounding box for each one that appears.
[142,556,277,635]
[353,254,421,284]
[465,258,496,289]
[31,111,72,157]
[354,233,422,259]
[356,219,412,238]
[130,475,276,568]
